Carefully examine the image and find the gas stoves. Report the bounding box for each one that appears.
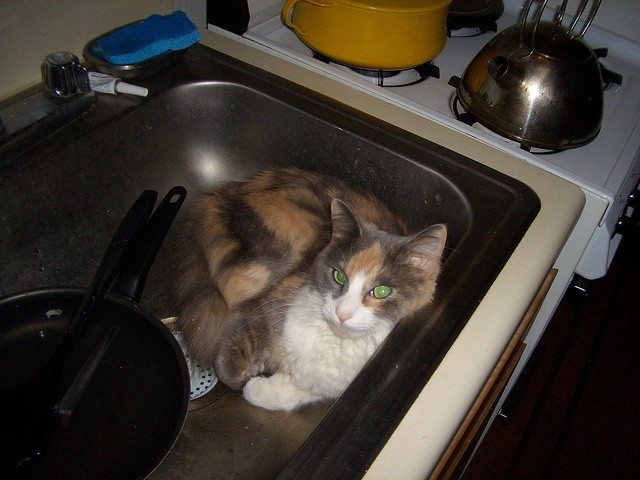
[239,0,640,280]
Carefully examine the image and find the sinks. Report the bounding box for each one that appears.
[0,81,538,479]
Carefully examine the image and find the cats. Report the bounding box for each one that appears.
[167,163,449,412]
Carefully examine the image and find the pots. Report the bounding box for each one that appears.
[280,0,452,71]
[0,185,191,480]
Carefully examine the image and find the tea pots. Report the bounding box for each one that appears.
[455,0,604,150]
